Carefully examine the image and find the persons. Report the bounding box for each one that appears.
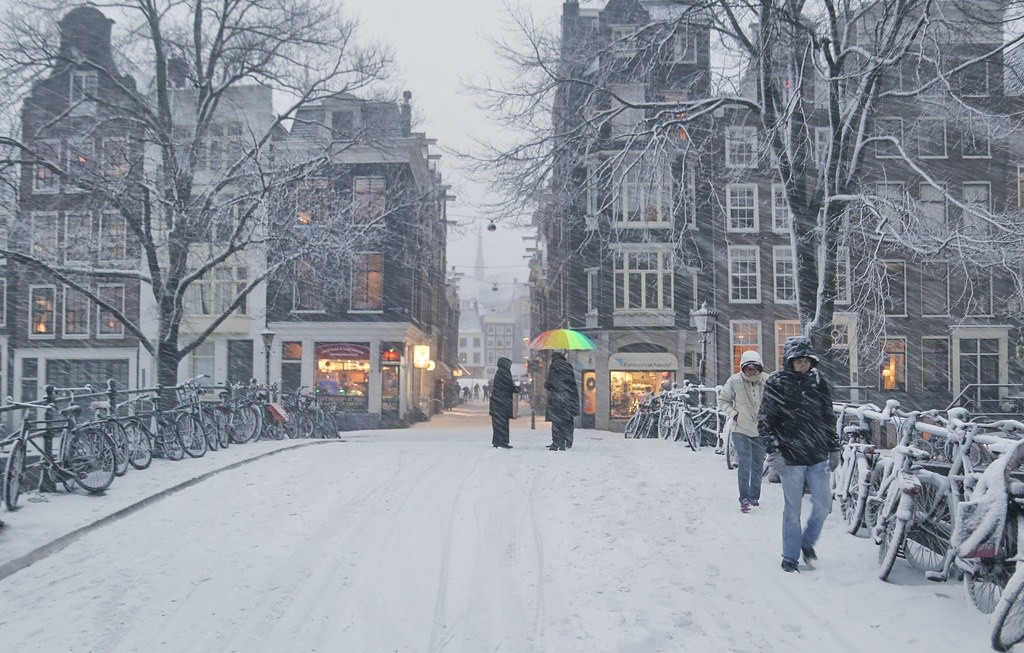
[489,357,519,449]
[758,337,841,572]
[543,354,580,451]
[717,351,772,513]
[444,379,532,412]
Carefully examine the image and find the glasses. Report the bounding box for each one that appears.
[744,367,758,371]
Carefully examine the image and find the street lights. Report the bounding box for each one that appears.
[692,299,718,406]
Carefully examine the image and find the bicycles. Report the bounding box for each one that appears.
[4,396,118,511]
[43,374,342,476]
[834,398,1024,651]
[725,417,770,475]
[623,378,699,452]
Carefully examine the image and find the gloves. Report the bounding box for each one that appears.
[734,414,738,422]
[768,449,786,475]
[829,452,839,472]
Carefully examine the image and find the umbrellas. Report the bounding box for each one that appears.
[529,330,597,353]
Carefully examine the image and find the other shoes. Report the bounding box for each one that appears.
[802,546,819,568]
[782,560,799,575]
[545,444,572,451]
[741,498,759,513]
[494,444,513,449]
